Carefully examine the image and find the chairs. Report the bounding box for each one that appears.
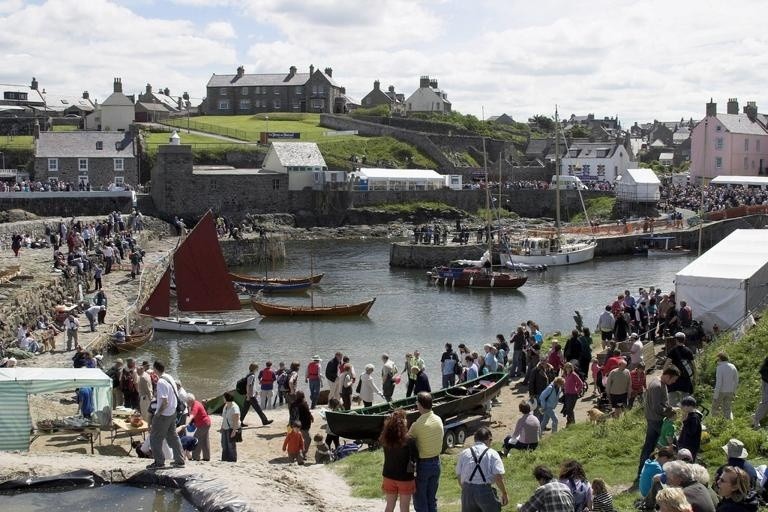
[23,296,86,354]
[174,412,190,435]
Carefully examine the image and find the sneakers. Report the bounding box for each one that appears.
[170,461,185,469]
[145,463,166,470]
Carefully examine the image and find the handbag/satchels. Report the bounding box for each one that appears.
[228,427,243,442]
[453,361,463,375]
[355,376,363,393]
[407,455,417,474]
[340,374,354,397]
[176,400,187,416]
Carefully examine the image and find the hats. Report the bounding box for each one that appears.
[310,354,322,361]
[722,438,748,459]
[630,333,638,338]
[672,332,685,339]
[676,448,693,464]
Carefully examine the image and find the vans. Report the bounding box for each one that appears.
[549,174,588,191]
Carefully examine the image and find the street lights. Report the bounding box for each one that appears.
[264,115,270,133]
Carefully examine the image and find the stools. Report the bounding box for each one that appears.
[179,436,198,460]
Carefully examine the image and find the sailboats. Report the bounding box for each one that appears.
[430,111,528,289]
[130,209,266,335]
[500,105,597,267]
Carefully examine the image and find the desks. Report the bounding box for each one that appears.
[108,405,153,459]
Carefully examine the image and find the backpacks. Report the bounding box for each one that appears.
[120,368,135,392]
[104,367,121,389]
[145,370,160,385]
[236,373,255,395]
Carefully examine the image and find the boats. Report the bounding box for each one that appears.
[504,259,549,272]
[633,234,676,258]
[327,369,506,441]
[647,240,691,259]
[168,238,379,331]
[113,324,153,350]
[150,388,246,417]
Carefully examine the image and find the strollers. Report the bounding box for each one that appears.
[330,439,362,462]
[672,405,709,455]
